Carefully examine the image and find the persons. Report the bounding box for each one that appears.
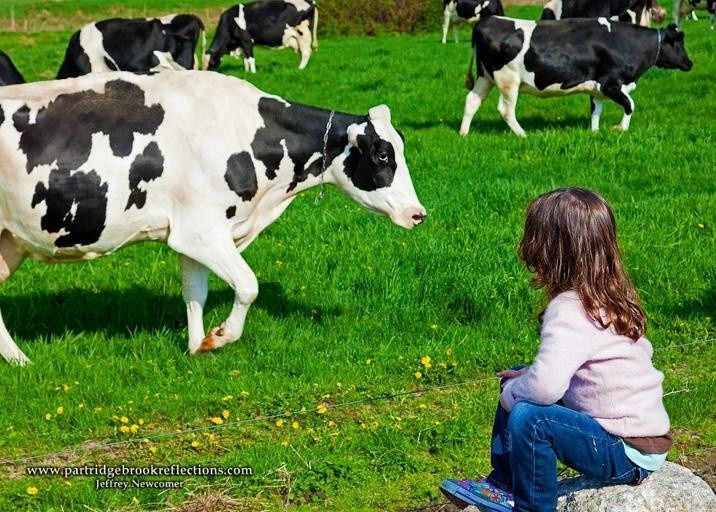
[439,187,674,512]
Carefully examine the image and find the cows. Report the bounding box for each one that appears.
[440,0,716,138]
[0,0,428,369]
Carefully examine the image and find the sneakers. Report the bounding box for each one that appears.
[439,479,515,512]
